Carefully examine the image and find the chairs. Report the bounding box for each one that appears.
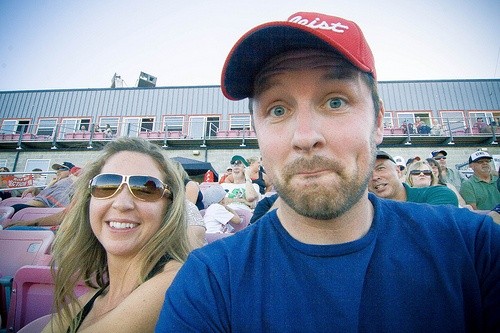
[200,180,277,243]
[0,194,108,333]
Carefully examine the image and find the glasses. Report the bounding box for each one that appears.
[477,118,483,120]
[435,156,446,160]
[230,160,242,167]
[55,167,67,171]
[88,172,173,201]
[409,170,433,176]
[477,159,491,164]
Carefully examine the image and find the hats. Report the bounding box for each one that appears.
[230,155,249,167]
[51,161,74,171]
[376,151,396,163]
[203,185,229,203]
[431,150,447,156]
[406,156,420,166]
[469,151,493,164]
[221,12,377,101]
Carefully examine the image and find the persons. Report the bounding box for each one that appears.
[366,151,458,207]
[155,13,500,333]
[95,125,101,132]
[459,151,500,215]
[427,158,465,205]
[41,134,192,333]
[402,116,442,134]
[104,124,112,138]
[393,156,420,182]
[405,160,435,188]
[488,167,500,224]
[0,162,82,230]
[431,149,468,189]
[479,117,500,133]
[173,155,279,250]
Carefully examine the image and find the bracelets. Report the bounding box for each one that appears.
[246,179,252,182]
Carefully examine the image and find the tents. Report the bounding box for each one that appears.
[171,156,218,182]
